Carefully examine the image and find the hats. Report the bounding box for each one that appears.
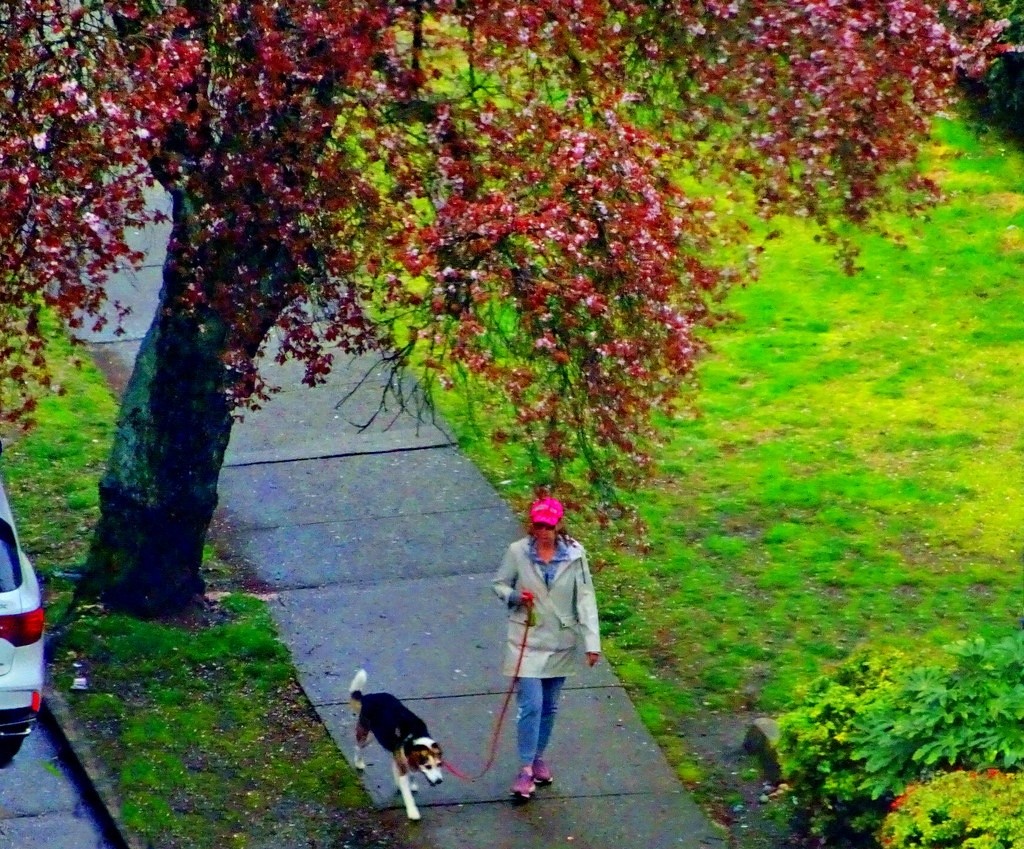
[531,497,563,526]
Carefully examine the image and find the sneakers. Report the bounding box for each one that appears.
[510,764,536,798]
[532,758,553,783]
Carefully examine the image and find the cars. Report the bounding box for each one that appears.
[0,487,45,768]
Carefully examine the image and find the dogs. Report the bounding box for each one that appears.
[347,668,444,824]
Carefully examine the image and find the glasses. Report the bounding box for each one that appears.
[532,522,556,530]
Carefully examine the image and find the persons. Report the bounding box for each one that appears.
[491,496,601,798]
